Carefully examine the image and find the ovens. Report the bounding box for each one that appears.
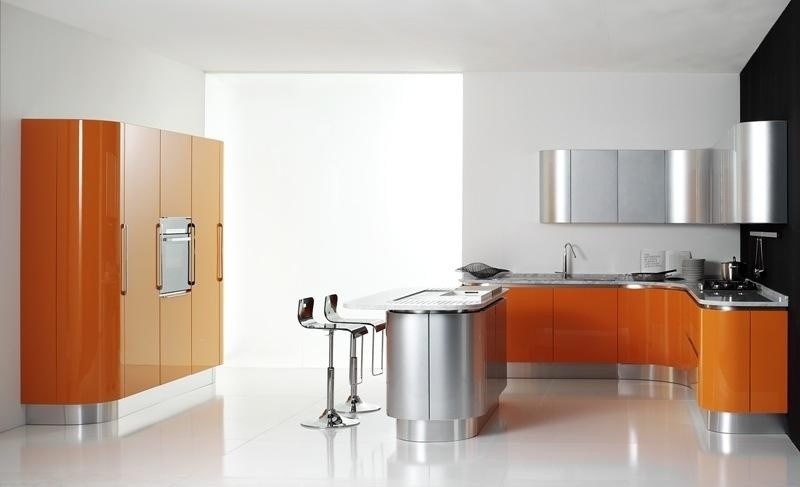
[159,216,193,297]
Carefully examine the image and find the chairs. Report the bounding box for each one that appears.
[324,294,388,414]
[297,297,370,430]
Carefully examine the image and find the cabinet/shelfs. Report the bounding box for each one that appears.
[538,118,790,230]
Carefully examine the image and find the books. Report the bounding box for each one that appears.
[439,289,501,303]
[451,285,502,298]
[639,248,692,276]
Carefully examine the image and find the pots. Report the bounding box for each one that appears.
[721,255,748,281]
[631,269,677,281]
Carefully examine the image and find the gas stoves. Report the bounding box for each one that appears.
[695,279,761,292]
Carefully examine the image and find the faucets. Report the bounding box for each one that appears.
[555,242,576,280]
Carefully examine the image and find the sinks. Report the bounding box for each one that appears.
[527,279,616,282]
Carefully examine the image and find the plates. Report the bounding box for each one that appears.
[681,258,706,281]
